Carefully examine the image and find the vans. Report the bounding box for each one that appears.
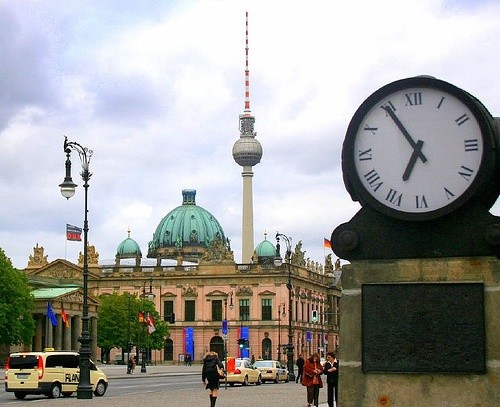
[4,348,108,400]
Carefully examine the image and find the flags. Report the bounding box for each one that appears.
[66,224,82,242]
[61,306,70,329]
[324,238,331,249]
[147,313,157,335]
[138,311,147,323]
[47,302,58,327]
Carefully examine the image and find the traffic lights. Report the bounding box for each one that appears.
[237,338,249,347]
[312,308,318,322]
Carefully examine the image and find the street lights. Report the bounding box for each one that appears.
[274,231,296,381]
[58,134,94,399]
[225,290,234,389]
[277,302,287,364]
[141,277,154,373]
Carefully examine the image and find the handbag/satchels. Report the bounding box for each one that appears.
[215,364,226,379]
[305,368,314,379]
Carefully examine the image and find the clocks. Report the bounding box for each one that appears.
[331,75,500,260]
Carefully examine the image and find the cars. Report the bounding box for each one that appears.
[220,358,263,387]
[254,360,290,384]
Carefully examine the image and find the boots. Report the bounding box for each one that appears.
[209,394,213,402]
[210,397,217,407]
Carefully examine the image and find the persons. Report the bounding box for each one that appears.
[201,351,224,407]
[295,354,304,384]
[323,351,339,407]
[188,354,192,366]
[130,355,136,374]
[301,353,323,407]
[185,355,188,366]
